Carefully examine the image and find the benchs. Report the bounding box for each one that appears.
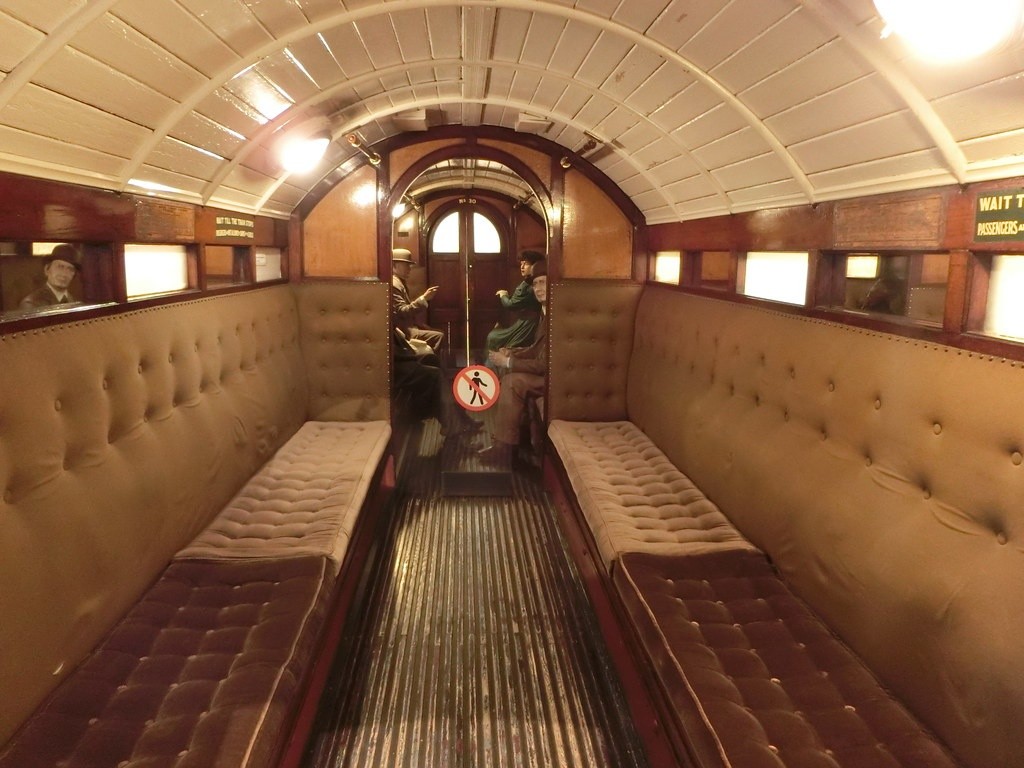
[544,280,1024,768]
[1,277,393,768]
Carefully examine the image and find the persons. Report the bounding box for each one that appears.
[478,250,547,459]
[870,256,908,315]
[393,249,483,436]
[20,244,85,310]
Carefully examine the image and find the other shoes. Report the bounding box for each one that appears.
[440,416,484,436]
[479,445,535,464]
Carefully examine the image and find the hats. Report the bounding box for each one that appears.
[525,260,548,285]
[393,248,418,266]
[43,245,84,273]
